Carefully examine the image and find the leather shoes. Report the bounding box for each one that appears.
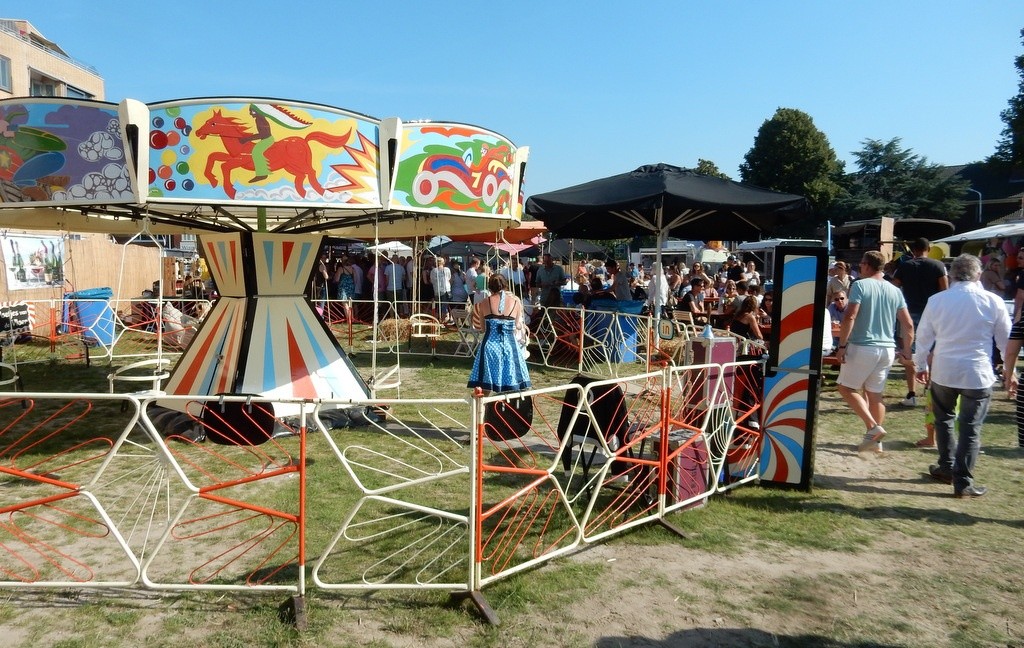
[954,486,987,498]
[930,464,953,485]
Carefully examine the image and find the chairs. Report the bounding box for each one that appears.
[452,309,481,356]
[557,375,651,523]
[672,310,701,334]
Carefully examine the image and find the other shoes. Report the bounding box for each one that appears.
[913,440,937,446]
[901,397,916,405]
[859,425,886,452]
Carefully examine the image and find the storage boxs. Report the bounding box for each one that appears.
[683,336,736,411]
[650,428,710,513]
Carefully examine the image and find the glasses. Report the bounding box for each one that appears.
[835,297,844,301]
[859,263,867,268]
[765,298,772,302]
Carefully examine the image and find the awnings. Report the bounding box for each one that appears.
[931,209,1024,243]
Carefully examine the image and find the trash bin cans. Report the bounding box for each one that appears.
[562,289,581,308]
[590,299,645,363]
[72,287,117,348]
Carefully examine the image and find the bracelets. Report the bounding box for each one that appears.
[838,345,847,349]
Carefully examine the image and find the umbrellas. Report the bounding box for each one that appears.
[543,238,608,290]
[366,240,414,259]
[525,163,807,354]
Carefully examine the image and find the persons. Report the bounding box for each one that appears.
[980,258,1006,298]
[882,263,904,292]
[914,253,1018,498]
[730,296,777,378]
[535,253,567,334]
[898,341,961,447]
[573,255,773,326]
[1002,301,1024,447]
[826,261,855,379]
[891,238,949,407]
[305,250,543,326]
[466,273,531,394]
[1015,247,1024,314]
[182,261,202,299]
[836,250,914,457]
